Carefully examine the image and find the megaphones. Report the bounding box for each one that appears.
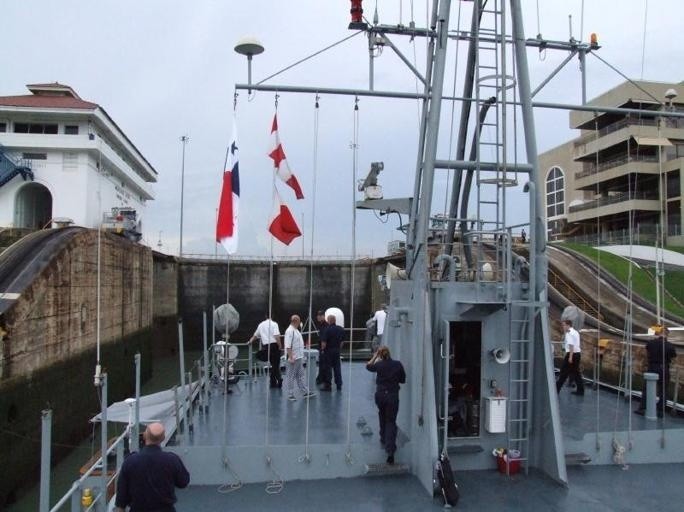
[386,261,406,289]
[465,263,493,279]
[492,346,510,365]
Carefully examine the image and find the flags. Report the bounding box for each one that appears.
[214,119,244,258]
[264,185,301,246]
[267,115,306,203]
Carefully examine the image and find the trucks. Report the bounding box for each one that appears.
[101,207,142,243]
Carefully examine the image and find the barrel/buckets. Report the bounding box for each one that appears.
[498,450,521,474]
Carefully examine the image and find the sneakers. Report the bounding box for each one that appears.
[315,375,342,391]
[381,437,394,465]
[270,379,283,389]
[633,403,664,416]
[571,391,584,396]
[287,394,297,401]
[303,391,317,399]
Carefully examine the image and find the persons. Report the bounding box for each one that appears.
[282,314,317,400]
[365,312,378,359]
[630,328,677,419]
[313,309,329,384]
[363,303,388,344]
[555,319,584,396]
[112,422,190,512]
[319,314,346,392]
[243,312,283,388]
[365,345,407,464]
